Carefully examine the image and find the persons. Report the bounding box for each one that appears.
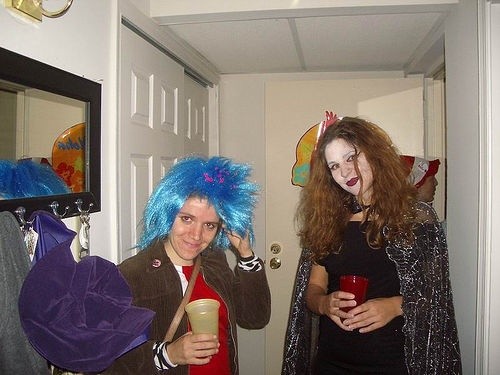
[279,116,465,375]
[101,154,272,375]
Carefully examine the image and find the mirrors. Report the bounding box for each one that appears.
[0,47,102,228]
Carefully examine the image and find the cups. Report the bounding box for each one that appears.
[339,275,369,324]
[184,299,220,342]
